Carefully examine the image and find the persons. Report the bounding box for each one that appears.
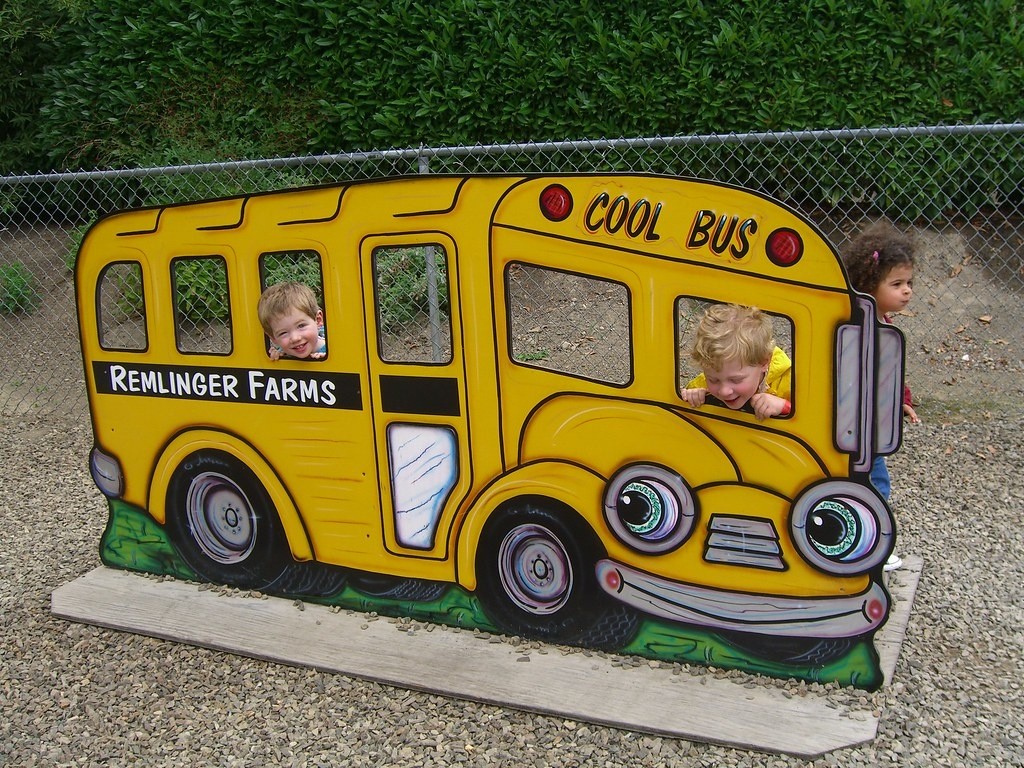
[679,302,792,422]
[841,219,921,570]
[257,282,326,363]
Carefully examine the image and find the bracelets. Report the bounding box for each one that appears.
[780,399,791,414]
